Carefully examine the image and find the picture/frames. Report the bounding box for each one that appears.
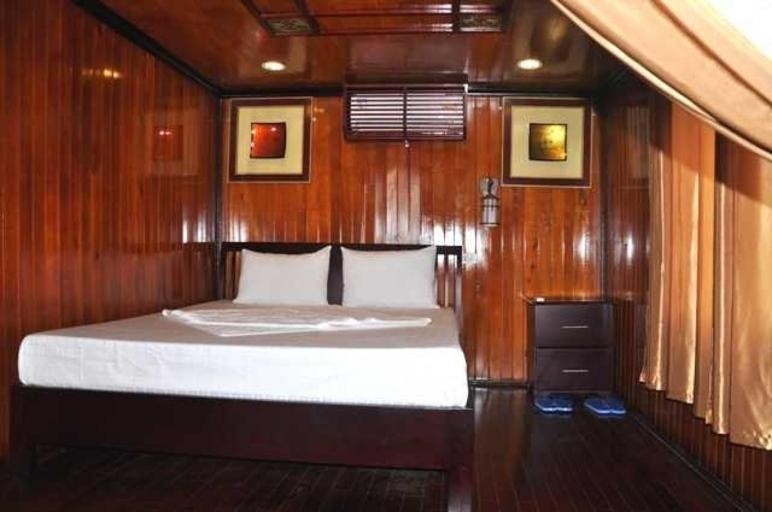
[143,106,203,176]
[229,97,313,181]
[500,96,594,190]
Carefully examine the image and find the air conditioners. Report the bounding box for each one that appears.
[343,84,468,141]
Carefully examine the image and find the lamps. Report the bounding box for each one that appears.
[481,176,499,225]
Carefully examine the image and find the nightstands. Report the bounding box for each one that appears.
[527,298,617,399]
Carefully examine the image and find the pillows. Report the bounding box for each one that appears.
[233,244,334,305]
[337,244,439,309]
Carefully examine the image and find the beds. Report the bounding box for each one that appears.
[12,242,476,512]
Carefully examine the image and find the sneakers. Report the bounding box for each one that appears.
[534,394,575,413]
[582,394,628,415]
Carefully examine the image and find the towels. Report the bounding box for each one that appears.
[162,308,432,338]
